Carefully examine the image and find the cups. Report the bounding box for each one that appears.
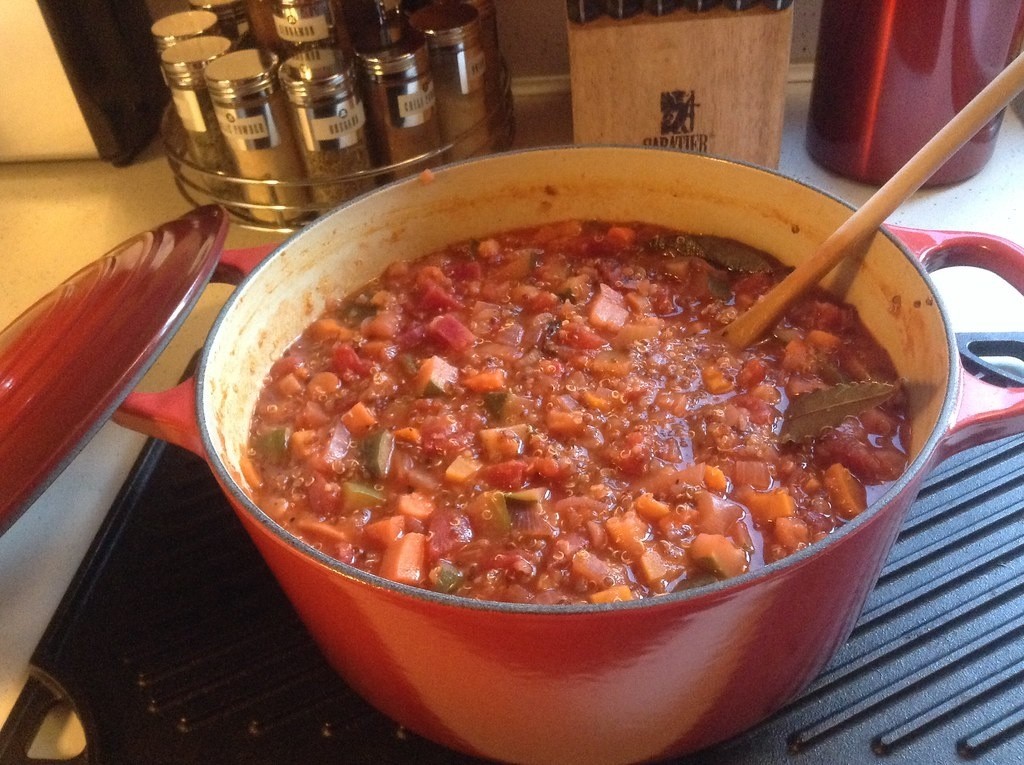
[806,0,1022,190]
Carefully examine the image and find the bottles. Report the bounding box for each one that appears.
[152,10,220,55]
[269,0,339,56]
[204,50,310,223]
[189,0,260,52]
[278,51,376,217]
[159,37,233,193]
[411,2,491,160]
[352,25,443,182]
[467,0,516,152]
[343,0,406,36]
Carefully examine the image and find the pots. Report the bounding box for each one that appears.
[112,143,1024,765]
